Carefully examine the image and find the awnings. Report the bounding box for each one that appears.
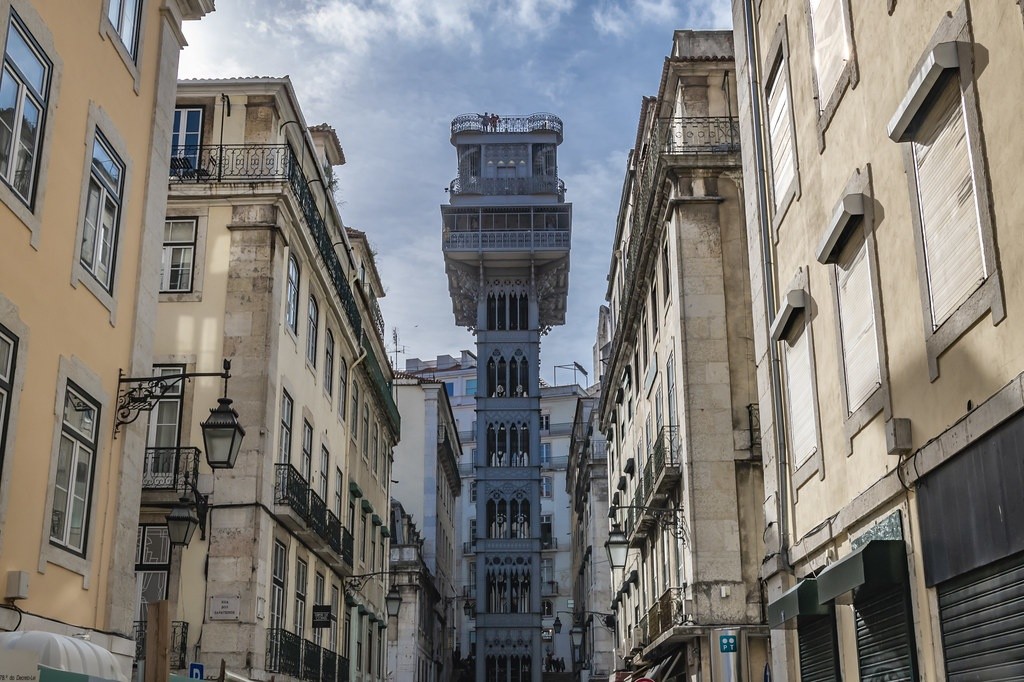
[0,630,122,682]
[817,539,906,606]
[767,578,833,631]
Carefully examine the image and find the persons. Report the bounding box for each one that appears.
[490,113,499,132]
[477,112,490,131]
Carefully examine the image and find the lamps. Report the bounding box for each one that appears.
[344,566,404,617]
[553,611,584,634]
[164,470,209,549]
[603,505,686,569]
[447,593,473,616]
[112,358,246,468]
